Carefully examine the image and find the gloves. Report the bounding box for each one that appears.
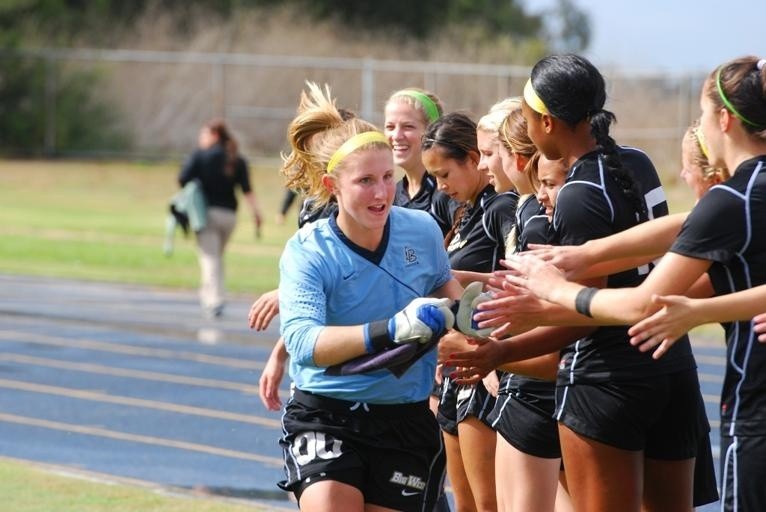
[363,281,495,363]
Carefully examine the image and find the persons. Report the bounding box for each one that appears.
[181,119,260,318]
[246,52,766,512]
